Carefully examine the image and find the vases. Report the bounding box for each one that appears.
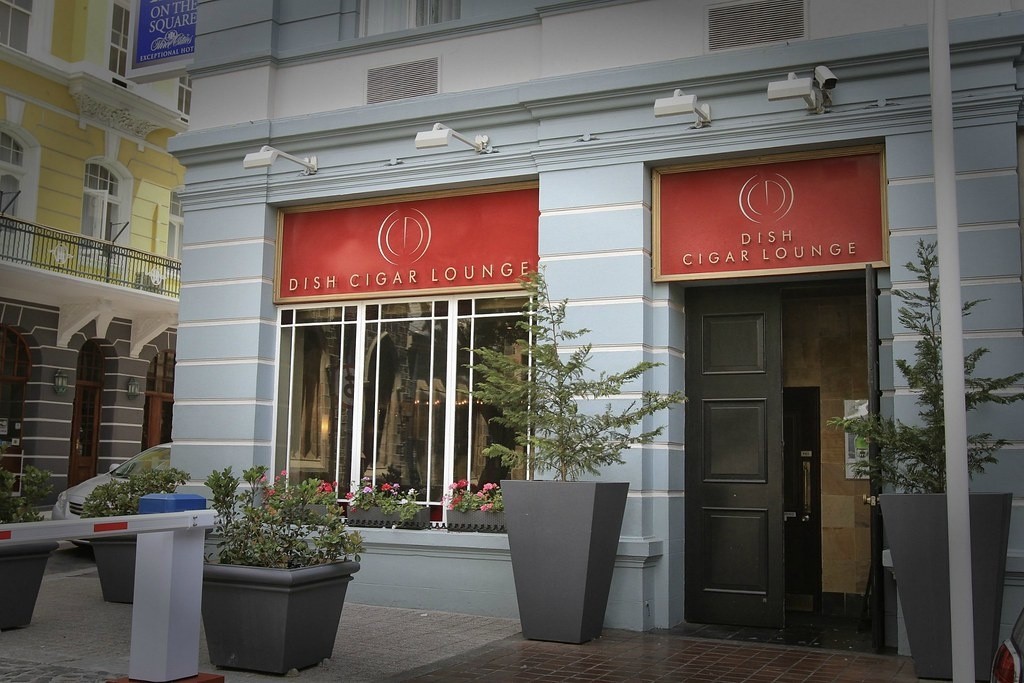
[347,504,431,529]
[261,502,330,525]
[444,510,507,533]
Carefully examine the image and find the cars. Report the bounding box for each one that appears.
[51,441,174,551]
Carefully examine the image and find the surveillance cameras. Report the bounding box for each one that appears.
[814,66,838,91]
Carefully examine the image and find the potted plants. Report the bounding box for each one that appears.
[0,443,60,629]
[80,468,192,603]
[826,237,1024,681]
[452,264,690,643]
[205,464,366,674]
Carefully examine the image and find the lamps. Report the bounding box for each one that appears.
[244,145,317,176]
[654,88,711,129]
[767,71,824,115]
[53,370,69,394]
[128,375,140,400]
[415,123,493,154]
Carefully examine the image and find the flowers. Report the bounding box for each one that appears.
[439,477,505,514]
[343,476,423,530]
[259,470,338,504]
